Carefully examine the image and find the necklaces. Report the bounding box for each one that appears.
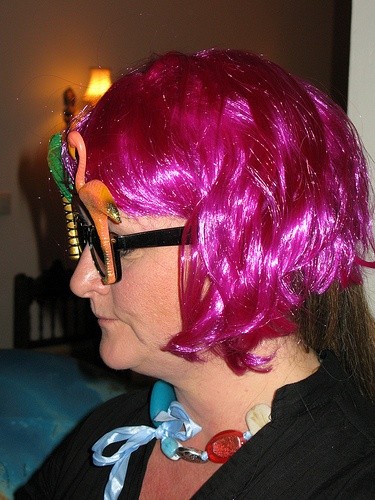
[150,378,272,463]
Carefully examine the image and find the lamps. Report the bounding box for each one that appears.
[82,67,113,106]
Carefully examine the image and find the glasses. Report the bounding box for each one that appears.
[45,128,194,286]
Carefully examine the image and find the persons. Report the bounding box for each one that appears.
[1,50,374,499]
